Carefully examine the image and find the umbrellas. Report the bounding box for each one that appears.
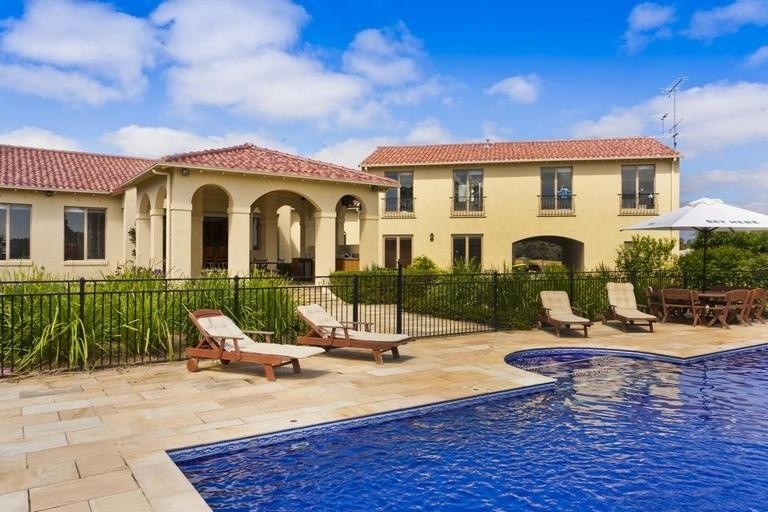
[619,196,768,293]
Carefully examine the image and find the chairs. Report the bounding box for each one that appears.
[535,281,768,339]
[181,302,415,382]
